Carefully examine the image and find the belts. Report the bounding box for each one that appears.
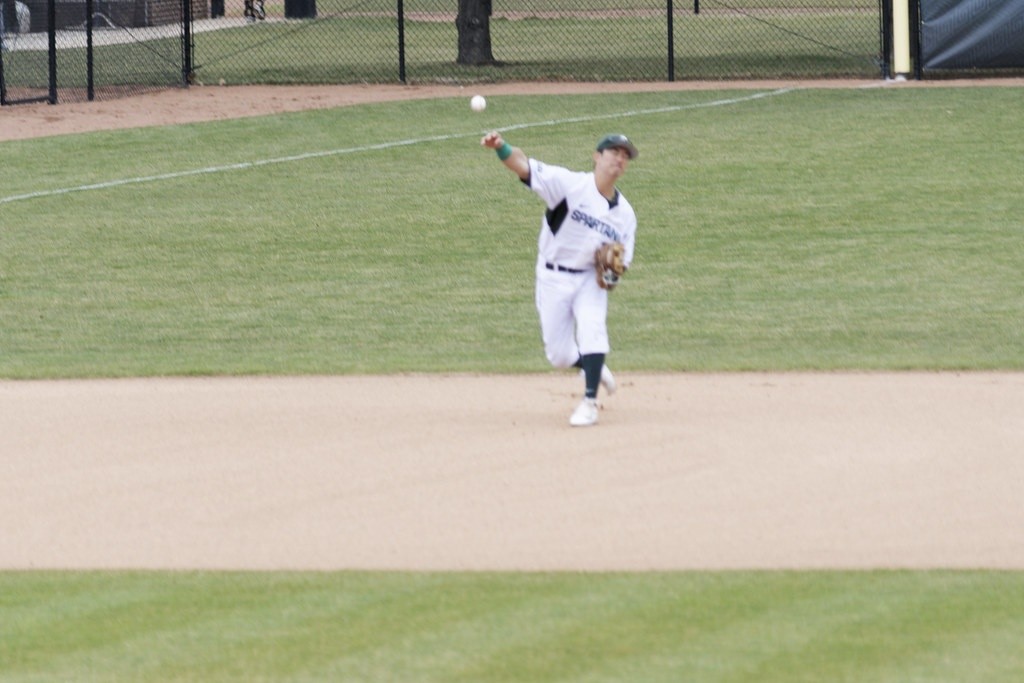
[546,262,586,274]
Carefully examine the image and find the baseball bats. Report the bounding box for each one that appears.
[594,241,626,291]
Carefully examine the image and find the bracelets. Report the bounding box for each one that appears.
[496,142,512,160]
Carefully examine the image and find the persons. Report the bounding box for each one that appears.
[480,130,637,426]
[243,0,266,23]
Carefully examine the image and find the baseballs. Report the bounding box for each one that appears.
[470,94,487,112]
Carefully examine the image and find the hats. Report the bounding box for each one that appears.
[598,134,638,160]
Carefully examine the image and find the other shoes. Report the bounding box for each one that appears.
[570,397,598,426]
[583,363,615,395]
